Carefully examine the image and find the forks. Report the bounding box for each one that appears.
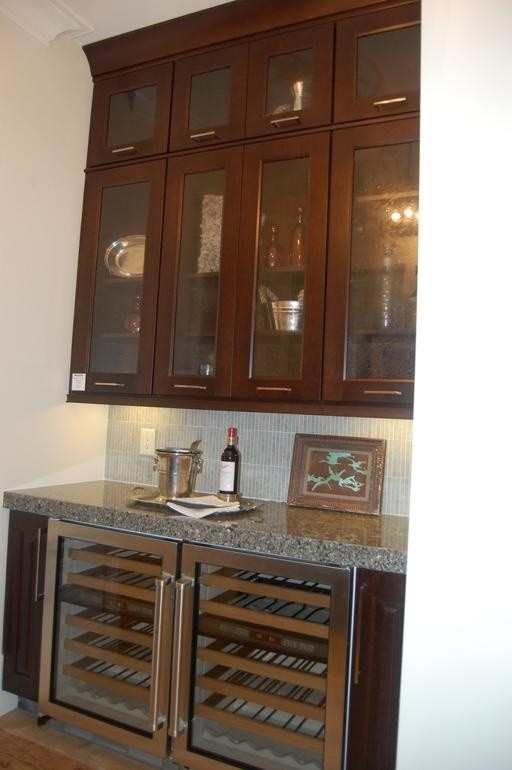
[188,440,202,453]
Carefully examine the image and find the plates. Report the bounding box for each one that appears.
[104,235,147,278]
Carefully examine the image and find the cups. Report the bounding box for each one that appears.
[290,79,303,112]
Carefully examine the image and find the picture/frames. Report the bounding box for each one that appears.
[287,433,387,517]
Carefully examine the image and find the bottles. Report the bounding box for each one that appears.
[123,296,143,335]
[219,427,239,493]
[374,242,416,330]
[265,207,304,268]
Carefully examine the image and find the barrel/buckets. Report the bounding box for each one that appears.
[153,447,204,500]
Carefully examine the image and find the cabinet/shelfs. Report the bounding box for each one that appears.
[66,0,418,419]
[37,518,358,770]
